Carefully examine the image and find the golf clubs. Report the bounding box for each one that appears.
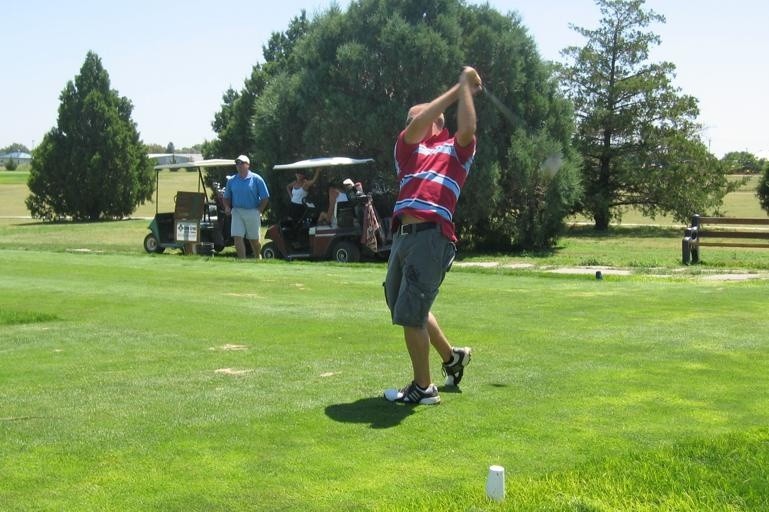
[372,181,390,194]
[477,82,563,180]
[213,181,227,197]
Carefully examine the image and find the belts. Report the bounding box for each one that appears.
[399,221,436,236]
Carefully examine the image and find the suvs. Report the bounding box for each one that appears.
[144,156,396,263]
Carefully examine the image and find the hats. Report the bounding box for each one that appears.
[235,154,251,165]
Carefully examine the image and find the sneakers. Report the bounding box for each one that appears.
[384,380,441,405]
[441,347,471,386]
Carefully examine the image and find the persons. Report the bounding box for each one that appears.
[223,155,270,258]
[344,178,356,200]
[317,183,349,225]
[383,67,481,405]
[287,167,320,213]
